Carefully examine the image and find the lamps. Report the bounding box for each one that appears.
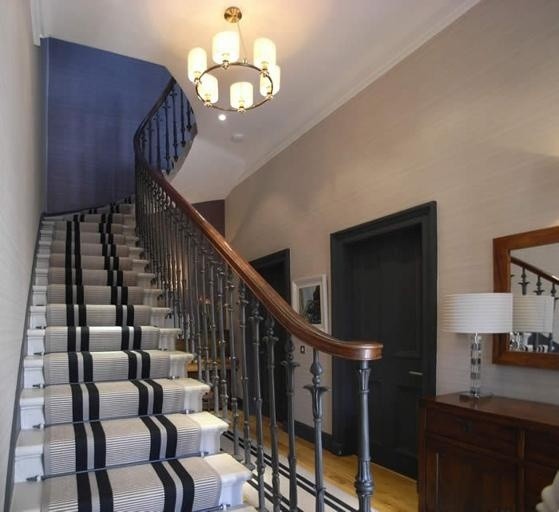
[187,5,282,116]
[510,295,555,351]
[441,290,514,403]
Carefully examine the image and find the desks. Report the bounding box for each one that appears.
[417,389,559,512]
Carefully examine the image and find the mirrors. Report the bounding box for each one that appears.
[492,224,559,371]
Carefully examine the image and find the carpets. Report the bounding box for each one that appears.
[41,193,237,512]
[218,415,377,512]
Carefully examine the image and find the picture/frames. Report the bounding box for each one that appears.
[290,272,329,336]
